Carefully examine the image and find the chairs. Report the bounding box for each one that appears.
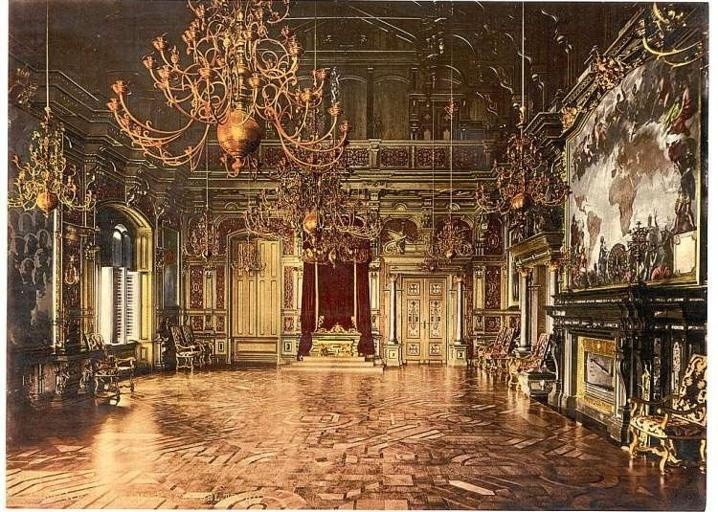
[170,324,213,375]
[84,332,137,407]
[619,352,707,476]
[477,325,552,392]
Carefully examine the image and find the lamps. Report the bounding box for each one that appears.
[104,2,395,268]
[418,2,477,281]
[478,2,570,240]
[8,1,102,220]
